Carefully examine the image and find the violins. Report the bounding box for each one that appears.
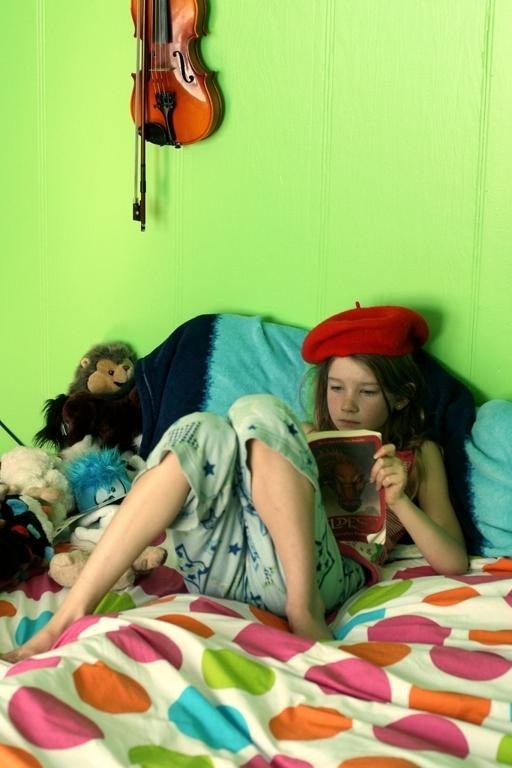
[127,0,225,152]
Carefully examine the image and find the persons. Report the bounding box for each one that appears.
[0,303,470,664]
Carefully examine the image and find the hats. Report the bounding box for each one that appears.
[301,301,430,364]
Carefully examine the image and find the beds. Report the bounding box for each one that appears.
[0,313,512,766]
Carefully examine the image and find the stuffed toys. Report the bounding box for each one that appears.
[0,339,166,598]
[1,446,167,591]
[35,340,138,470]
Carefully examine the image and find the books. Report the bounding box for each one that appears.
[303,430,388,546]
